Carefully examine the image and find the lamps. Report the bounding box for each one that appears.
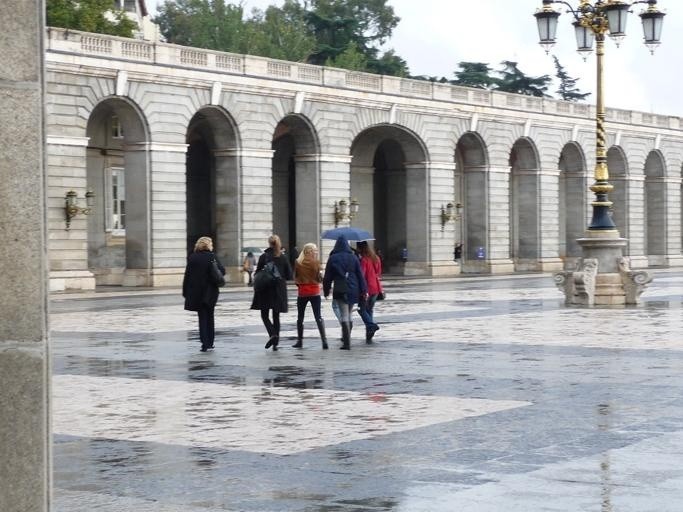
[439,200,464,232]
[65,187,98,230]
[333,195,359,227]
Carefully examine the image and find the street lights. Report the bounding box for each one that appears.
[534,0,665,238]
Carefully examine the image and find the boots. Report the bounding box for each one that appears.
[340,321,353,349]
[292,320,304,347]
[316,318,329,348]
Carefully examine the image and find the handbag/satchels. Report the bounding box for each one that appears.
[208,255,226,287]
[254,251,279,291]
[332,277,348,300]
[376,291,385,300]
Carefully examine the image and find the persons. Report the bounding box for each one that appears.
[355,240,382,344]
[291,243,329,351]
[243,251,257,285]
[323,236,368,351]
[182,237,225,353]
[249,234,292,352]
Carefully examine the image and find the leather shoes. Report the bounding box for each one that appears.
[366,324,379,340]
[265,334,279,351]
[199,344,215,352]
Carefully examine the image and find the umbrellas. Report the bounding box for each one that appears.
[320,226,376,254]
